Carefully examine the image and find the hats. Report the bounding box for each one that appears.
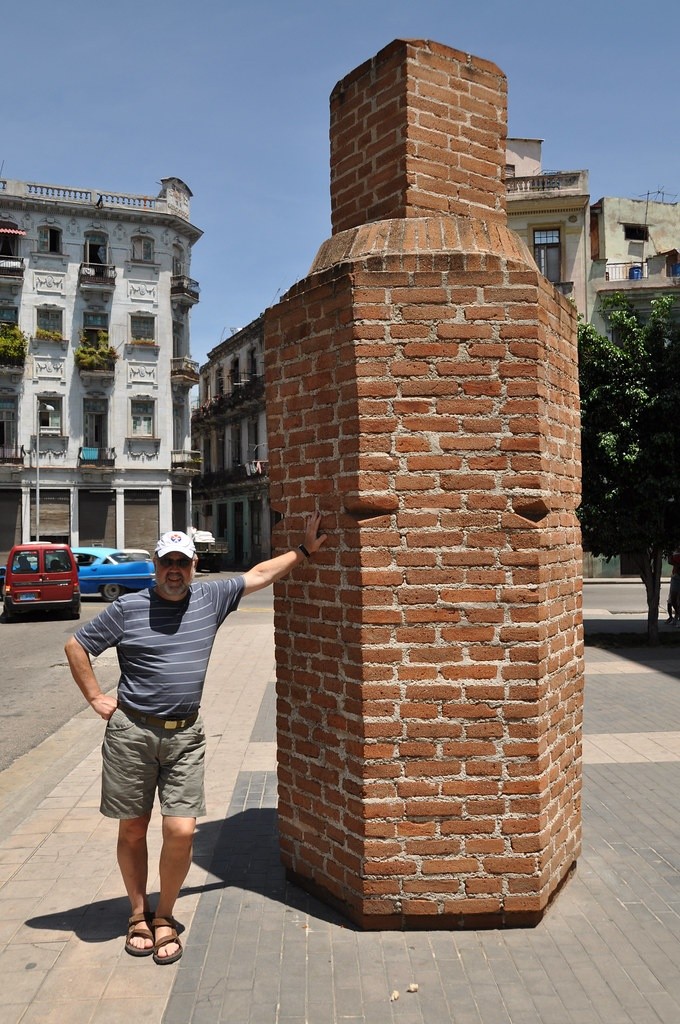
[154,531,196,559]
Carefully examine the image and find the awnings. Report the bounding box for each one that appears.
[0,228,27,237]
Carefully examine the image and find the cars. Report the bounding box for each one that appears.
[122,547,152,562]
[0,547,156,601]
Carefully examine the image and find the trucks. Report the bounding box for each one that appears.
[193,540,230,573]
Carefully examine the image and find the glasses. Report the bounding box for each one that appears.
[159,557,191,569]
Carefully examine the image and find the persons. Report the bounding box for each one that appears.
[15,556,34,571]
[664,565,680,626]
[63,512,330,969]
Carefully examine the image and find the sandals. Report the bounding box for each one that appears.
[152,915,183,965]
[125,911,155,955]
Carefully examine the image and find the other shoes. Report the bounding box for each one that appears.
[672,618,680,627]
[665,616,673,625]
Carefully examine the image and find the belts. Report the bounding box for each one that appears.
[117,704,199,730]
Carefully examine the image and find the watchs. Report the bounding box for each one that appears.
[297,543,310,558]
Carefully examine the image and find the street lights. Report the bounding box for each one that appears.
[35,400,55,541]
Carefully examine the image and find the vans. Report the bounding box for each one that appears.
[3,543,82,620]
